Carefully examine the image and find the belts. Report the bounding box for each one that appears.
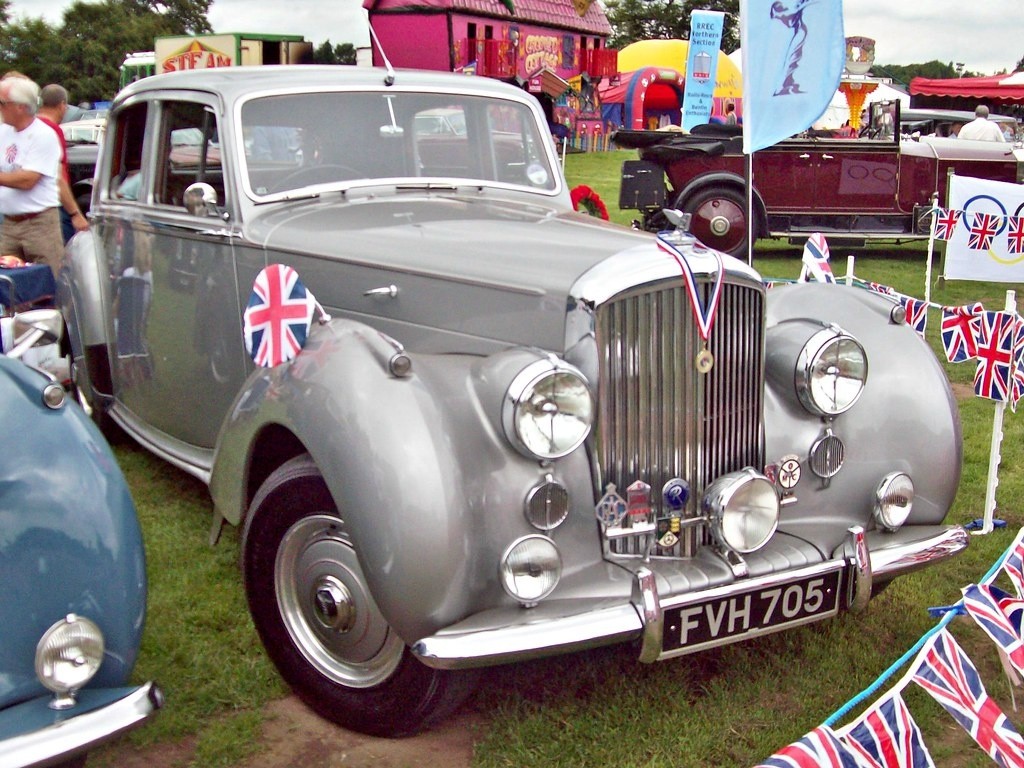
[3,207,61,222]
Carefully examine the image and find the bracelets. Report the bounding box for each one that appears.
[69,210,82,217]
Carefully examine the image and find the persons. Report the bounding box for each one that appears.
[186,188,208,212]
[877,108,894,126]
[0,71,65,310]
[836,108,867,137]
[100,219,157,384]
[725,104,737,125]
[956,104,1006,143]
[948,121,964,139]
[33,82,90,245]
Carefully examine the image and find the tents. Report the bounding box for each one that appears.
[909,67,1024,120]
[594,38,742,129]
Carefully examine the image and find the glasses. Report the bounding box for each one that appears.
[0,97,6,107]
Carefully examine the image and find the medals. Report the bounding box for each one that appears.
[694,345,715,375]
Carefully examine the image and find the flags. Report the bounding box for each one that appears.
[934,207,1024,254]
[799,229,1023,413]
[739,0,846,156]
[753,524,1024,768]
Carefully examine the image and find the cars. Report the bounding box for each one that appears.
[167,126,225,170]
[606,100,1024,258]
[58,118,104,219]
[0,352,161,768]
[49,64,971,741]
